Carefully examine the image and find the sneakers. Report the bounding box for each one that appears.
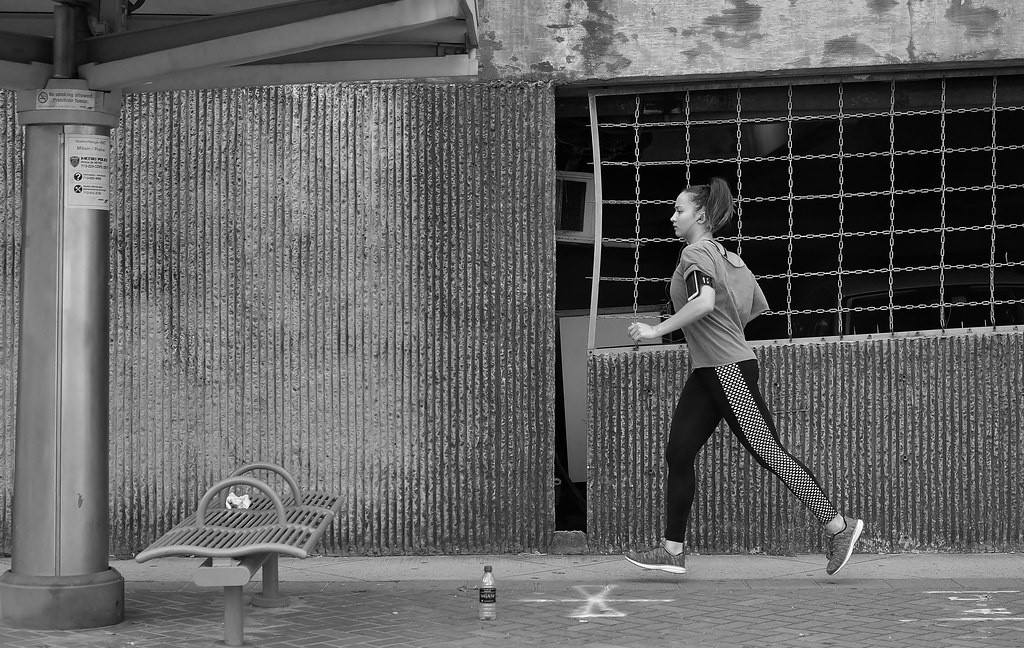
[624,546,686,574]
[825,515,863,576]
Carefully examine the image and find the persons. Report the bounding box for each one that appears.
[623,177,865,578]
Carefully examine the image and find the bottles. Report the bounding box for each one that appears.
[479,565,497,621]
